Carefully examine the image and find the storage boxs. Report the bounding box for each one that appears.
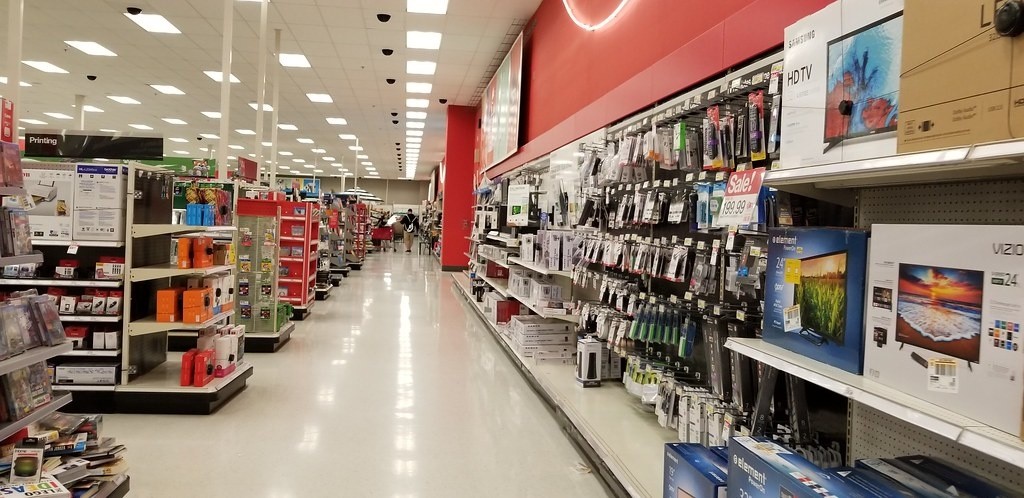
[856,458,970,498]
[896,0,1024,153]
[470,230,795,447]
[864,223,1024,437]
[727,434,866,498]
[0,139,306,498]
[826,466,906,498]
[897,454,1013,498]
[779,0,903,171]
[663,442,728,498]
[763,225,870,376]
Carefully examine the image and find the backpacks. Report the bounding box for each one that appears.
[405,215,417,232]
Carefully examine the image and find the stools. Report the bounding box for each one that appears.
[419,242,432,256]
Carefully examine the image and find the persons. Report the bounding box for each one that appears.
[400,208,419,252]
[370,211,392,251]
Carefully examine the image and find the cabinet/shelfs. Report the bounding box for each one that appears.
[239,181,322,321]
[167,177,296,352]
[330,222,352,278]
[30,161,256,417]
[450,0,1024,498]
[286,195,334,302]
[339,207,363,271]
[354,203,371,261]
[0,185,73,443]
